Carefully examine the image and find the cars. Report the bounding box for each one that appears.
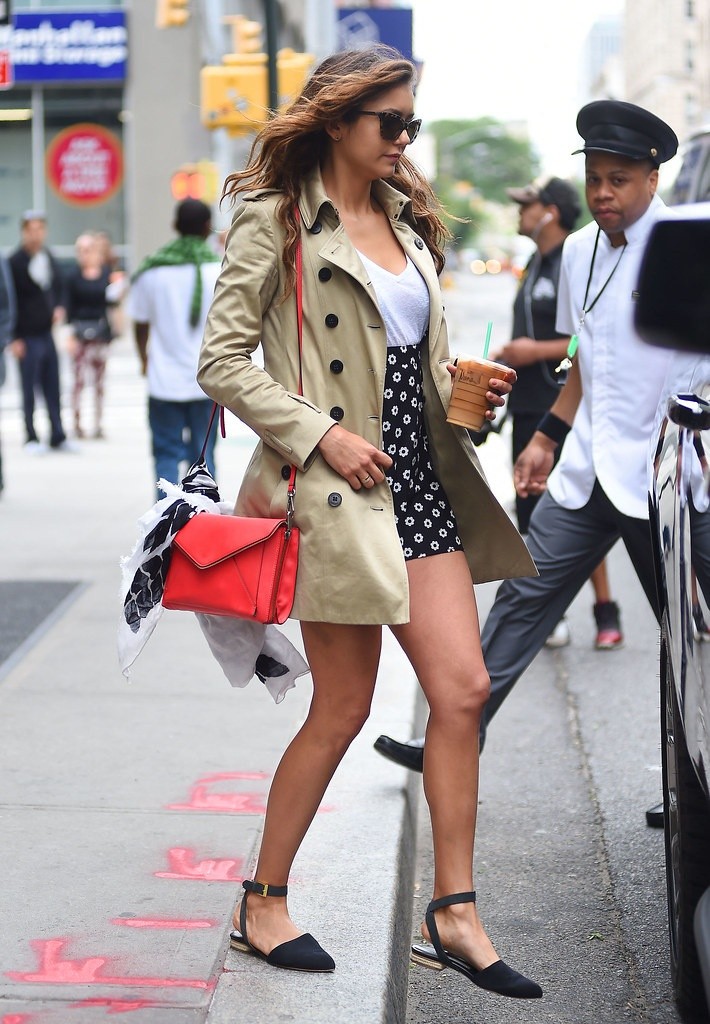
[628,204,710,1024]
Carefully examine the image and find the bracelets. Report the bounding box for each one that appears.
[537,411,571,445]
[693,437,705,457]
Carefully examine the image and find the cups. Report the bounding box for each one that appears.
[446,352,510,432]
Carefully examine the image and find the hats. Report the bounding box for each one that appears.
[506,174,583,230]
[17,210,45,228]
[571,98,678,169]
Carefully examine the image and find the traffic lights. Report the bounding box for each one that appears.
[156,0,190,29]
[231,14,265,53]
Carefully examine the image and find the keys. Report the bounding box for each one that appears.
[555,358,572,373]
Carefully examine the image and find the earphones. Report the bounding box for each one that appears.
[542,212,553,224]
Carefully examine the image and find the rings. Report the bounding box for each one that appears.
[361,475,371,484]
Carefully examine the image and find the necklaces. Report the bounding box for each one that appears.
[568,227,629,358]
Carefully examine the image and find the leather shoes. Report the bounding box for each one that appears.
[645,803,663,825]
[375,734,426,772]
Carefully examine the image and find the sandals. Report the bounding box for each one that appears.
[410,891,546,1000]
[229,878,336,973]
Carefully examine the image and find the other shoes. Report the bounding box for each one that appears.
[547,617,570,645]
[590,600,623,647]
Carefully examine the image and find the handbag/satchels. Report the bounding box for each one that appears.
[161,513,297,626]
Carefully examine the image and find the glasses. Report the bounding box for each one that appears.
[344,111,422,146]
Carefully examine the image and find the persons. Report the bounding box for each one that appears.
[487,175,625,649]
[130,199,224,501]
[196,43,543,1000]
[654,418,710,641]
[372,99,680,828]
[8,210,127,454]
